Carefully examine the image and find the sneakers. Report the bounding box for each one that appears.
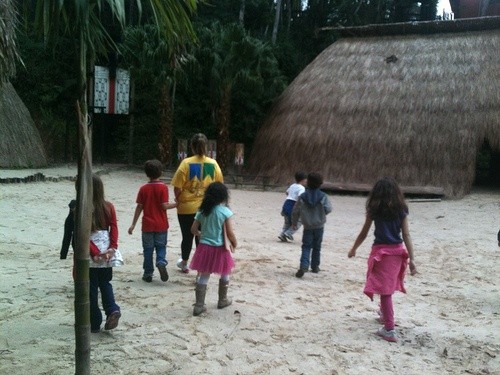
[377,326,398,342]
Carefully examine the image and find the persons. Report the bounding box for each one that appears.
[59,174,121,333]
[277,170,308,242]
[348,176,417,342]
[170,133,224,273]
[191,181,237,316]
[128,159,179,282]
[291,172,333,277]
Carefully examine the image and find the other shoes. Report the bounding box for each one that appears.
[92,326,102,333]
[176,257,191,274]
[157,263,170,282]
[377,315,385,324]
[295,268,308,278]
[141,275,153,283]
[103,310,121,331]
[277,232,288,242]
[283,231,295,240]
[311,267,321,273]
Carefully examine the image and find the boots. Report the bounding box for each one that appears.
[217,278,233,309]
[192,283,208,316]
